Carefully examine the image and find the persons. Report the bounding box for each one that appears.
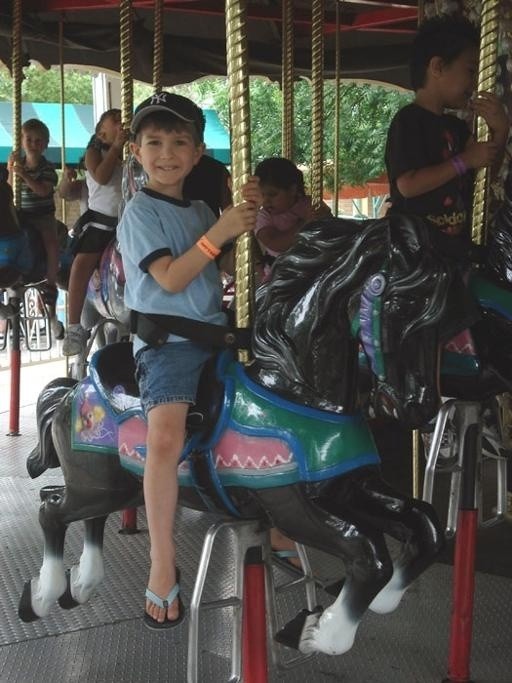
[113,90,264,631]
[382,10,508,473]
[0,106,331,360]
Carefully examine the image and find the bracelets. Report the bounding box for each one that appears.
[195,234,222,260]
[448,154,467,177]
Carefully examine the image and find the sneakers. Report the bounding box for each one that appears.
[60,323,90,357]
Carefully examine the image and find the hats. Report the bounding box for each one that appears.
[128,88,202,137]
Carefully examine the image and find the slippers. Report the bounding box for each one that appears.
[140,564,190,633]
